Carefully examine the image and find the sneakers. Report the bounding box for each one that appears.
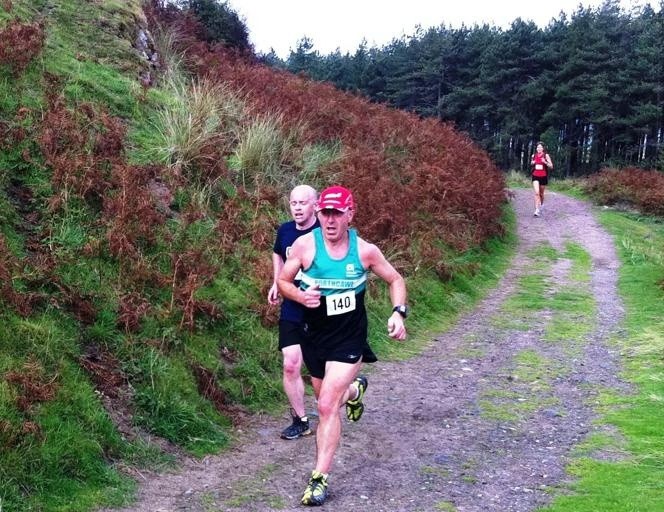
[280,416,311,439]
[534,203,544,216]
[302,470,329,505]
[345,375,368,425]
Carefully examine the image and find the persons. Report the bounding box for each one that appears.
[530,141,553,217]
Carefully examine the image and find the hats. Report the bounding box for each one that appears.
[315,186,354,213]
